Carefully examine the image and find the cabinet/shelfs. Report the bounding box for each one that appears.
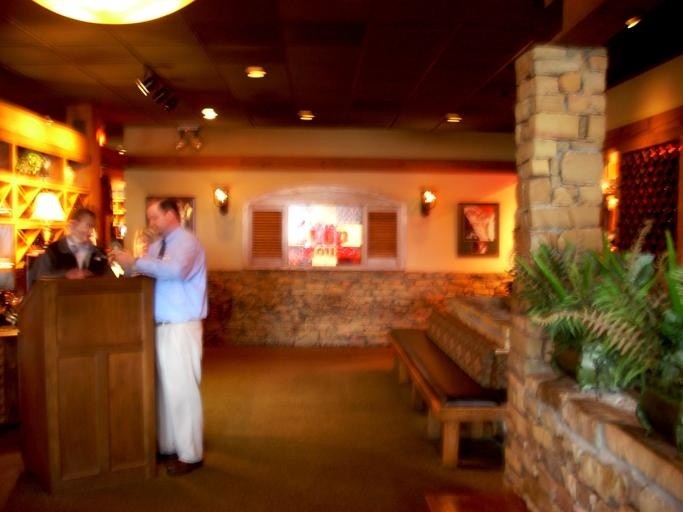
[0,101,125,340]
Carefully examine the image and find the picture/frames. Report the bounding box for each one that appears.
[456,201,499,258]
[144,196,196,237]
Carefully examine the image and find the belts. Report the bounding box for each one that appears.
[153,320,174,328]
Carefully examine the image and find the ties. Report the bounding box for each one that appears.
[152,240,166,287]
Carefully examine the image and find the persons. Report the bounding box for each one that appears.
[104,194,209,477]
[26,210,122,281]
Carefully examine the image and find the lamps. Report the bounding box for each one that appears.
[214,187,228,215]
[175,123,204,150]
[419,186,436,217]
[133,71,175,110]
[32,193,67,249]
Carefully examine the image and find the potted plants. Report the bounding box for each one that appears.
[503,232,682,452]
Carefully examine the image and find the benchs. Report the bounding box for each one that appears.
[388,329,508,471]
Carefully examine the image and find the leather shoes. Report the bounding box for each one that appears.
[156,449,204,476]
